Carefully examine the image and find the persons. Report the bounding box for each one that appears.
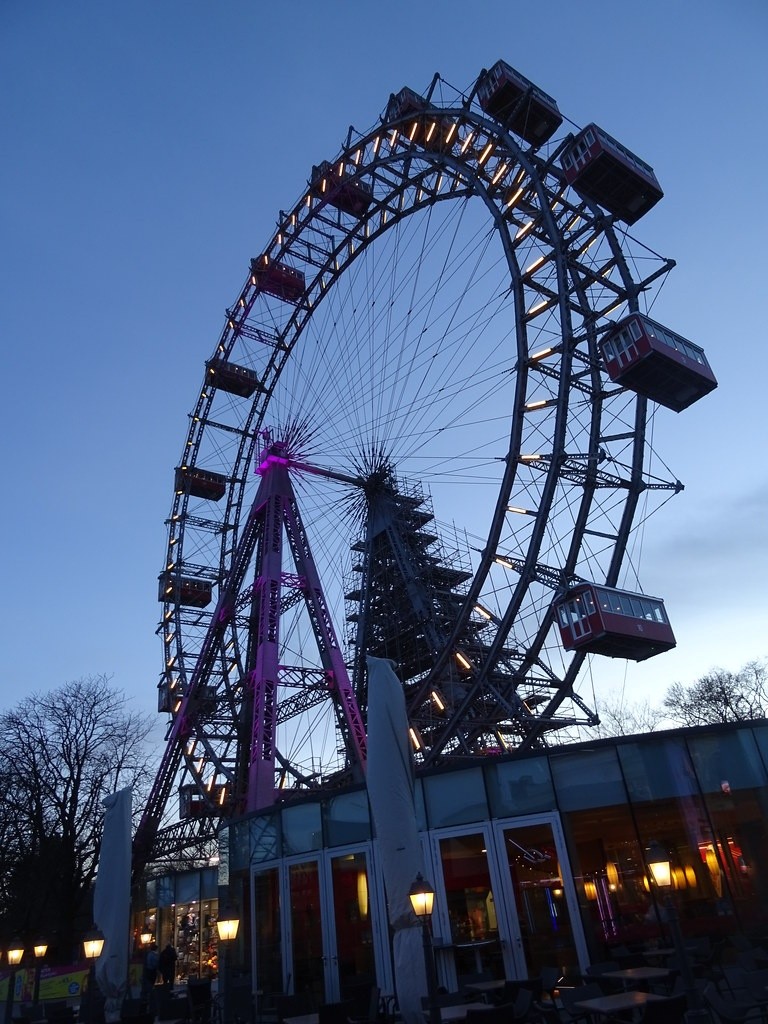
[639,893,680,929]
[141,943,177,1004]
[180,907,211,963]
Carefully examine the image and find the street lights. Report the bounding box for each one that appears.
[78,923,106,1024]
[405,871,447,1024]
[32,936,49,1024]
[139,925,153,1024]
[3,936,25,1024]
[213,902,241,1024]
